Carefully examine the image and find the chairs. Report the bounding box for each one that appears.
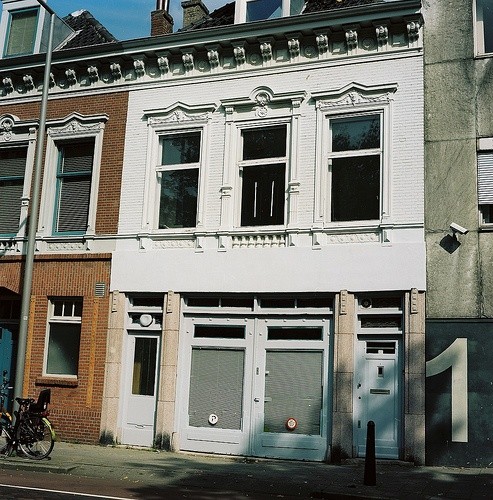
[28,387,51,432]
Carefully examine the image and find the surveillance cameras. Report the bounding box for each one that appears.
[450,222,470,238]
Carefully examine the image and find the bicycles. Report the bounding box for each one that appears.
[0,370,57,460]
